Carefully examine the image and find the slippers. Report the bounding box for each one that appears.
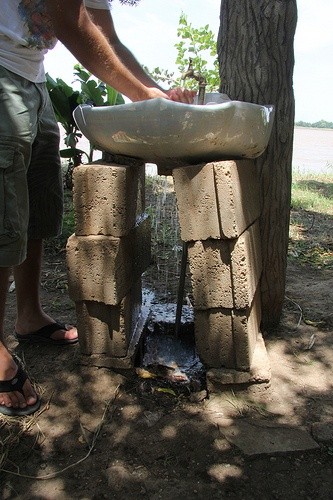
[0,366,41,416]
[15,319,78,342]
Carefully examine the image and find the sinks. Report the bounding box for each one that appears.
[72,92,277,160]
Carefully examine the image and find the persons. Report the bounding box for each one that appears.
[1,0,195,417]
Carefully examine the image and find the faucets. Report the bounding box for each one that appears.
[181,58,197,81]
